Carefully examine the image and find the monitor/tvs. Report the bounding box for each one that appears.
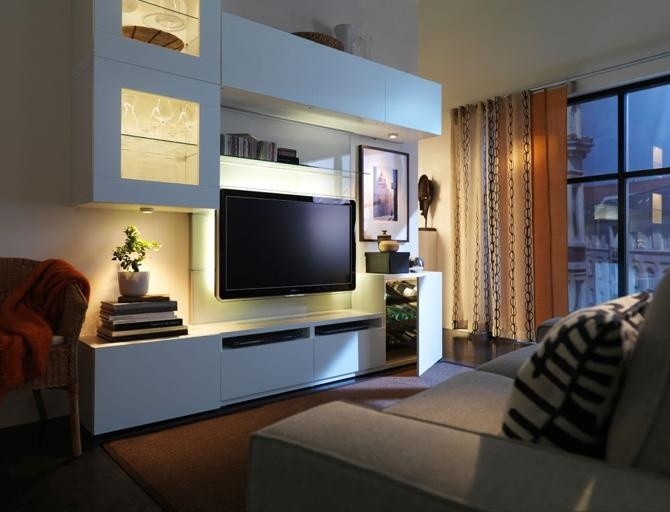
[218,188,357,299]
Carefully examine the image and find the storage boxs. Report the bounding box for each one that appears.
[365,252,410,274]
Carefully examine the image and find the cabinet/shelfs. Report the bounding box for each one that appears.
[67,0,220,214]
[313,313,385,388]
[219,322,313,405]
[354,271,443,377]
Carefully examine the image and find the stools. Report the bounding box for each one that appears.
[0,257,90,458]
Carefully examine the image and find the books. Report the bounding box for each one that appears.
[219,132,299,165]
[96,298,187,340]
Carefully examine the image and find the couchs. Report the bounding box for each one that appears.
[248,267,670,512]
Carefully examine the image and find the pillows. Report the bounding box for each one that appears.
[501,289,655,460]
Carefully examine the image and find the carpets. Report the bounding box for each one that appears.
[100,359,477,512]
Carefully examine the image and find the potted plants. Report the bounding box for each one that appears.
[111,224,162,296]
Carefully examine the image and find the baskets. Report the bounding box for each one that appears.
[121,23,186,51]
[295,32,343,51]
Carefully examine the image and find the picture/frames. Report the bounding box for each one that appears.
[359,144,409,242]
